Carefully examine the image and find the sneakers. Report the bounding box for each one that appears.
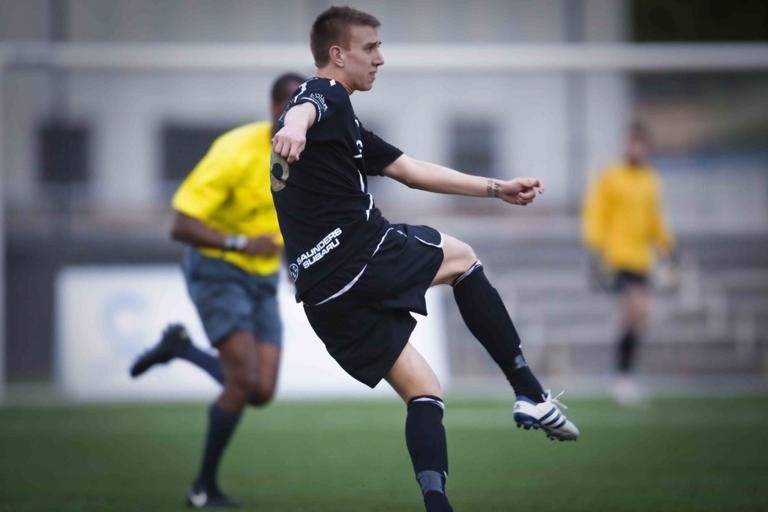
[132,324,186,376]
[513,389,580,441]
[186,483,240,509]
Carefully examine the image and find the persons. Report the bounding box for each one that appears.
[582,120,679,408]
[270,5,581,510]
[131,73,308,507]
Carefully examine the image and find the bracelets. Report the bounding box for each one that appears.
[225,234,247,251]
